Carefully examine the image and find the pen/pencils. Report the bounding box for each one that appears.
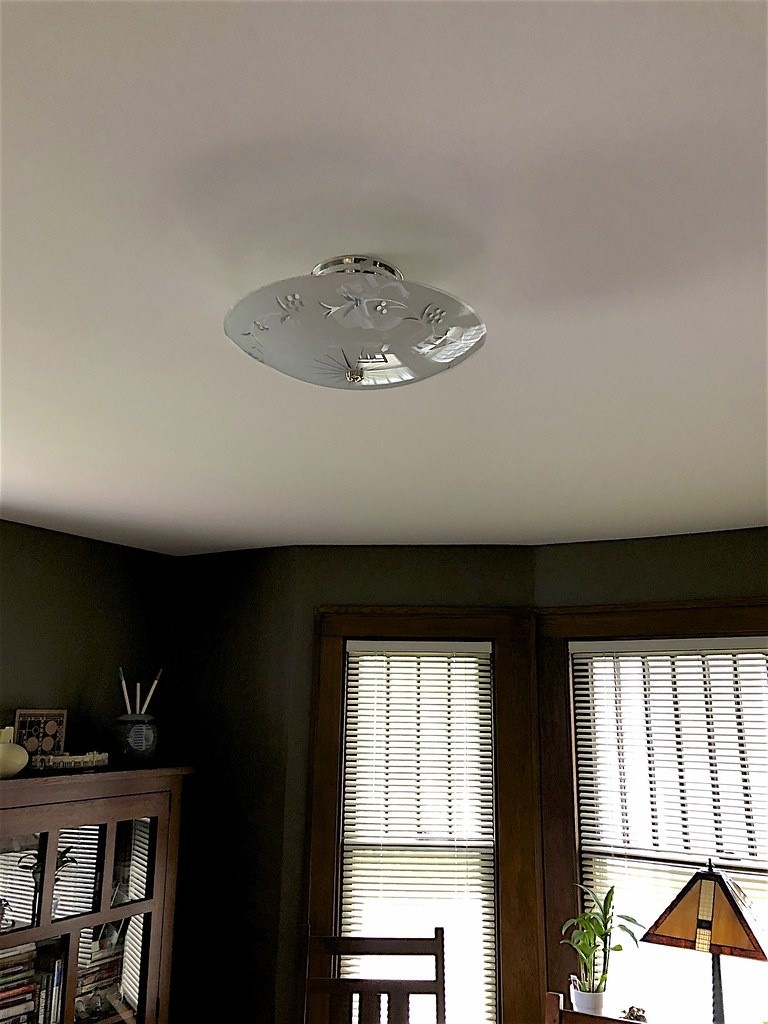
[136,683,140,714]
[118,667,131,715]
[141,669,163,713]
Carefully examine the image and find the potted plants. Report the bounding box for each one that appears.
[560,883,646,1015]
[17,846,77,890]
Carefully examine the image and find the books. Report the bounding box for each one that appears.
[1,939,63,1024]
[75,958,124,993]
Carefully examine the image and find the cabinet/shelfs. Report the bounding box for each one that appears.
[0,759,198,1024]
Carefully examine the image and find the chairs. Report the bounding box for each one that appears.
[289,923,446,1024]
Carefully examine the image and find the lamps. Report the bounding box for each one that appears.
[223,255,488,390]
[639,858,768,1024]
